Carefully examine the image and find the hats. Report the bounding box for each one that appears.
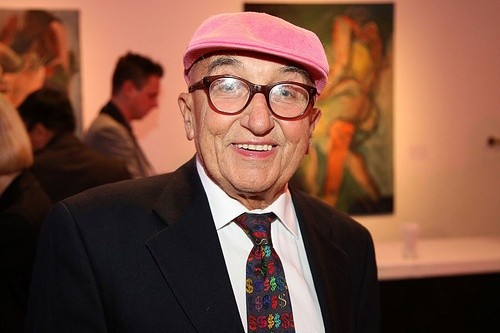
[181,12,330,97]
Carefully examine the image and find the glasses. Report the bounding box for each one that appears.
[188,75,318,121]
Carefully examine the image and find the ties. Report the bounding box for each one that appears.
[233,213,297,333]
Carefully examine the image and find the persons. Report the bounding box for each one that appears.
[0,92,53,333]
[84,50,164,179]
[62,12,383,333]
[16,88,133,204]
